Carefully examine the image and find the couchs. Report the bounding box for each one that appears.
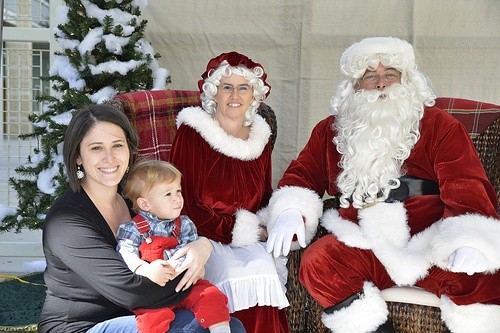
[116,90,500,333]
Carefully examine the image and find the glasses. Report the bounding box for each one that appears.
[361,72,401,81]
[218,85,250,94]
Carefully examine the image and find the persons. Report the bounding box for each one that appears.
[266,36,500,333]
[167,51,288,333]
[36,103,246,333]
[116,160,231,333]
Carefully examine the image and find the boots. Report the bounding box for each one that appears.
[322,281,394,333]
[440,295,500,333]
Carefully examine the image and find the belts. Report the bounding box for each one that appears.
[388,177,440,201]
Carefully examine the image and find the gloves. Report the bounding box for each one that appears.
[446,246,488,276]
[265,209,306,258]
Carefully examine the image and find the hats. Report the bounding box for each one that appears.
[341,36,415,62]
[197,51,271,109]
